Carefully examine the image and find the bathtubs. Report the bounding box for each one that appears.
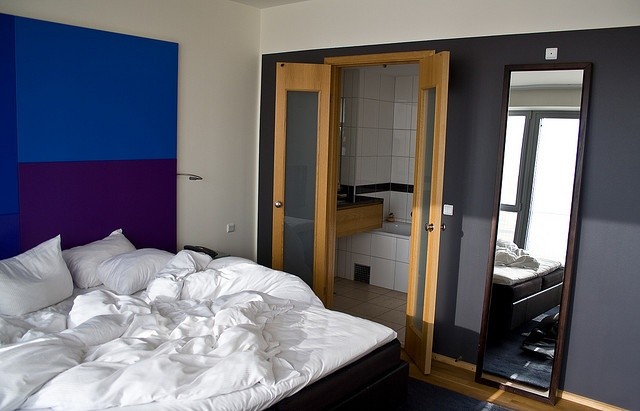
[377,221,411,239]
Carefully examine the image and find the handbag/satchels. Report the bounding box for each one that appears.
[521,323,559,361]
[538,313,559,338]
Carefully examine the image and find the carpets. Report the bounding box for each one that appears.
[372,377,511,410]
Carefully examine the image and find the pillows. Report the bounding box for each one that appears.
[85,248,176,295]
[61,228,136,289]
[0,233,74,316]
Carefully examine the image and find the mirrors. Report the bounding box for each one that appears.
[473,61,594,406]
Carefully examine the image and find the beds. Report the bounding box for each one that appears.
[489,237,565,332]
[0,237,410,411]
